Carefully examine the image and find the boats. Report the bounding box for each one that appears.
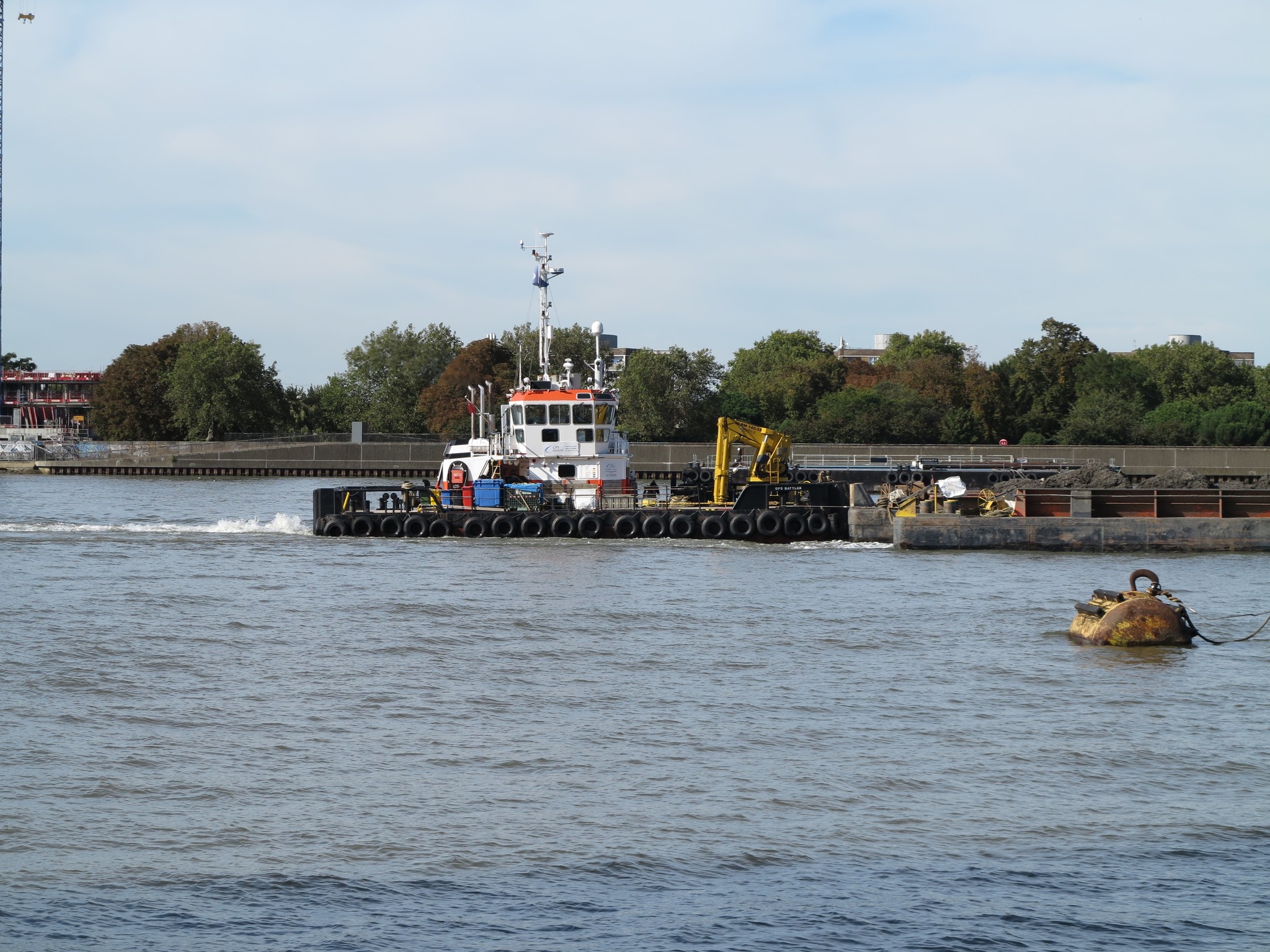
[312,229,850,543]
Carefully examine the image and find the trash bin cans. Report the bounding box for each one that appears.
[461,486,475,511]
[451,488,464,512]
[441,488,453,511]
[429,488,442,512]
[473,479,506,508]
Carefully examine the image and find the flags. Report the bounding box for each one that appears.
[468,400,476,416]
